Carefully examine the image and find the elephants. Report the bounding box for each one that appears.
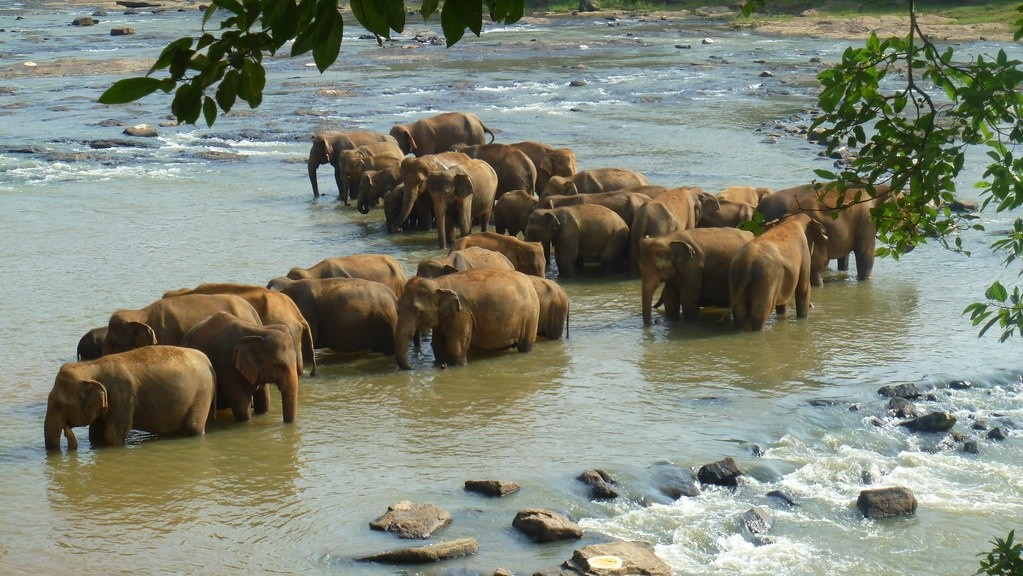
[44,111,917,455]
